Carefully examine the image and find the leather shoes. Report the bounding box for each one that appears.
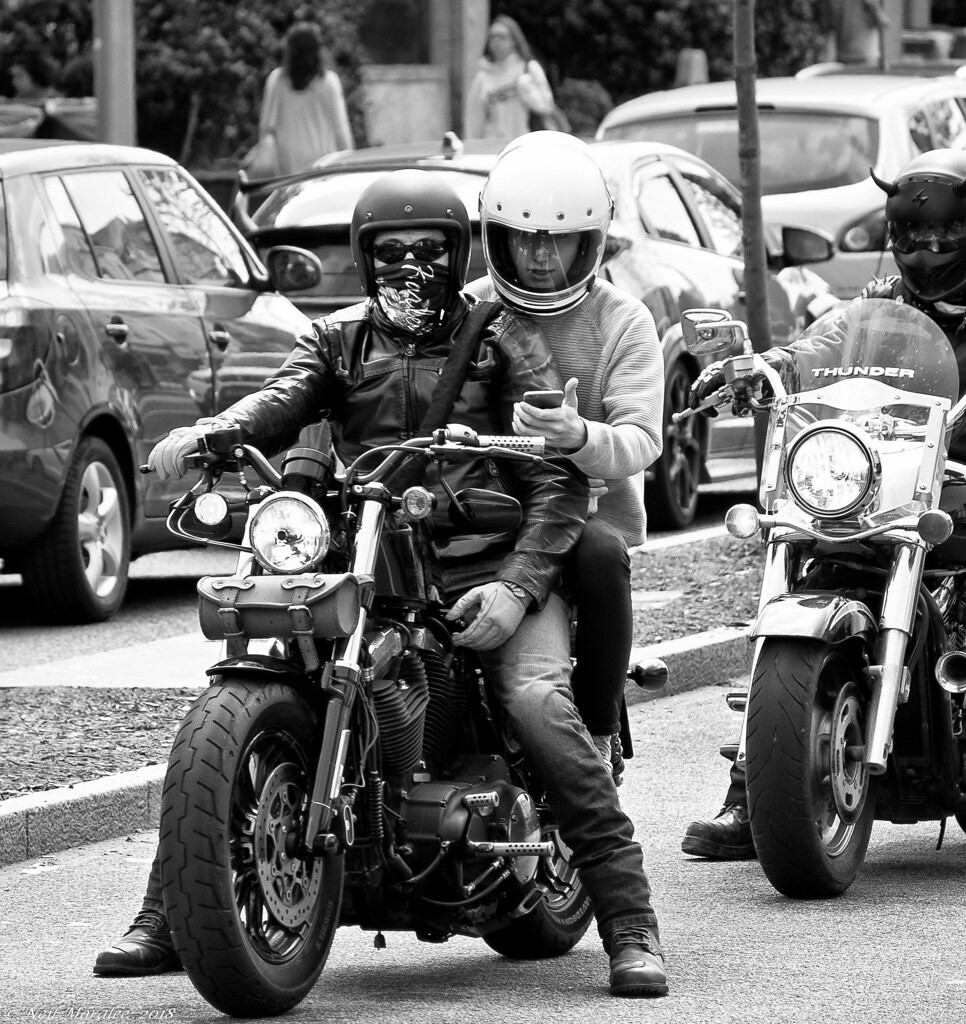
[94,909,179,975]
[610,926,670,994]
[682,803,756,860]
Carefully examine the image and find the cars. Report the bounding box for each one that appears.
[0,139,323,623]
[228,57,966,529]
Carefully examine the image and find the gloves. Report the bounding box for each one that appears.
[445,580,532,649]
[146,419,231,482]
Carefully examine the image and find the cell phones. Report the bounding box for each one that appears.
[523,390,564,411]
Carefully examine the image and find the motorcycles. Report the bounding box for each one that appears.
[140,423,669,1018]
[672,296,966,900]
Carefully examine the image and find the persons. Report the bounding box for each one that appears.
[466,16,554,136]
[462,128,663,784]
[8,54,66,139]
[95,168,669,995]
[682,146,966,862]
[259,23,354,170]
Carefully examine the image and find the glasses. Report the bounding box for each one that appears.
[364,237,455,265]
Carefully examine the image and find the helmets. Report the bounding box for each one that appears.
[869,148,966,302]
[350,168,472,295]
[477,131,614,317]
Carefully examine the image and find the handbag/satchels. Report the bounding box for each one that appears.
[239,130,281,181]
[530,104,572,135]
